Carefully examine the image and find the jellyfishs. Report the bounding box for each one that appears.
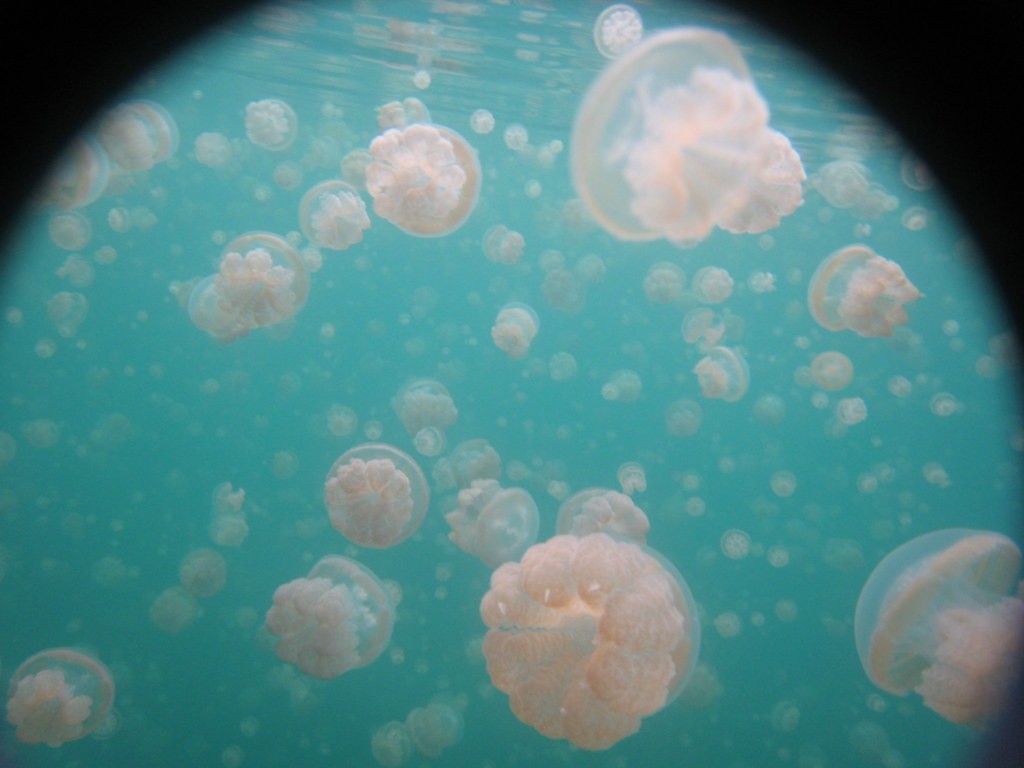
[0,5,1023,768]
